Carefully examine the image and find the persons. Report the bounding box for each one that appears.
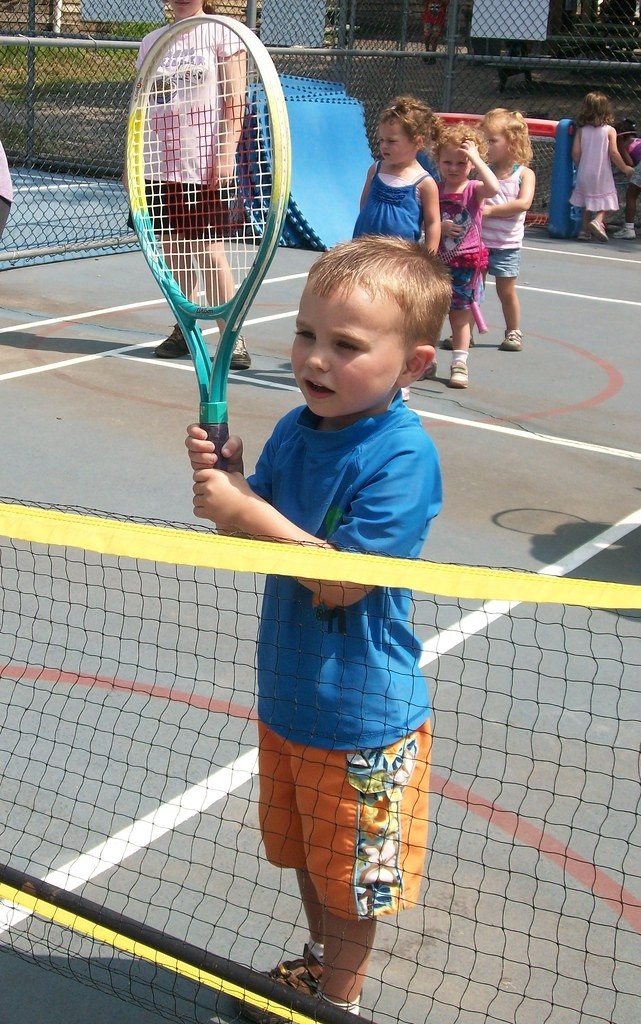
[612,118,641,239]
[421,0,450,65]
[568,91,634,242]
[353,95,441,400]
[119,0,253,369]
[186,235,454,1024]
[419,120,501,389]
[445,107,536,352]
[495,40,534,96]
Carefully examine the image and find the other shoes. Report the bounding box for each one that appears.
[429,59,436,64]
[423,58,428,61]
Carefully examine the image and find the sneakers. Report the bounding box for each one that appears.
[613,227,636,238]
[449,360,468,387]
[500,330,524,351]
[230,335,251,367]
[402,386,410,401]
[238,943,362,1022]
[416,360,437,381]
[443,335,474,348]
[589,219,609,242]
[578,230,592,239]
[155,322,203,357]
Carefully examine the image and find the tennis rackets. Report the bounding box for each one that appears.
[123,13,291,470]
